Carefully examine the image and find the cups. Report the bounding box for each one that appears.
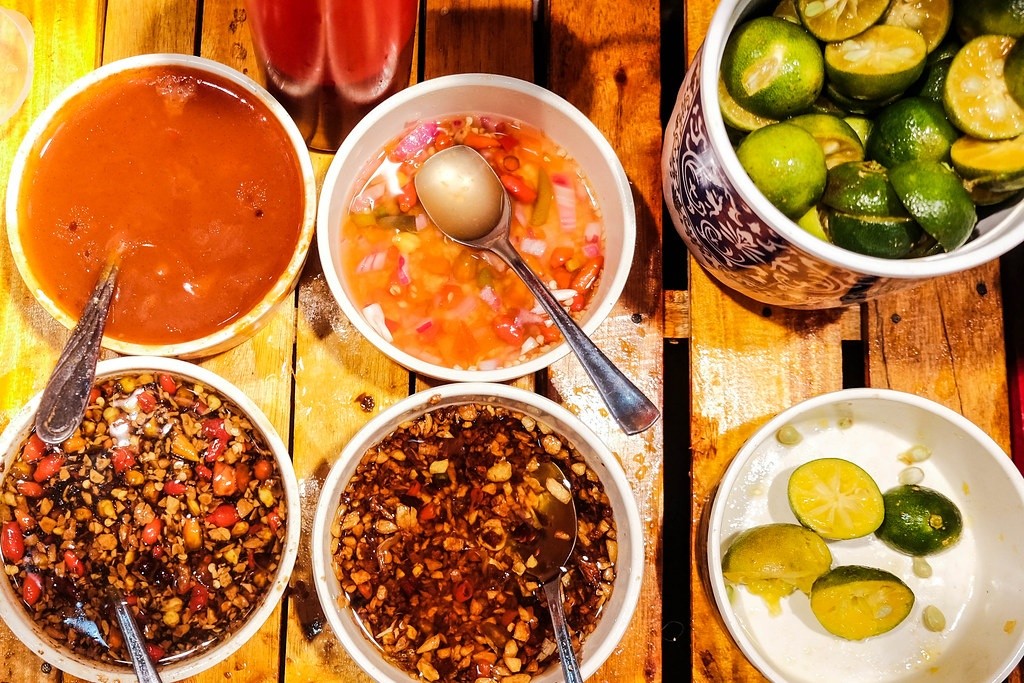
[661,0,1023,310]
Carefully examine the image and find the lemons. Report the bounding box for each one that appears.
[717,0,1024,259]
[721,458,963,639]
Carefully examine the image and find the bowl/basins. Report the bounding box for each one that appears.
[0,355,300,683]
[311,383,645,682]
[316,73,638,380]
[5,51,315,358]
[708,387,1024,682]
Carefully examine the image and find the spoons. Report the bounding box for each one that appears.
[525,460,588,683]
[415,144,661,435]
